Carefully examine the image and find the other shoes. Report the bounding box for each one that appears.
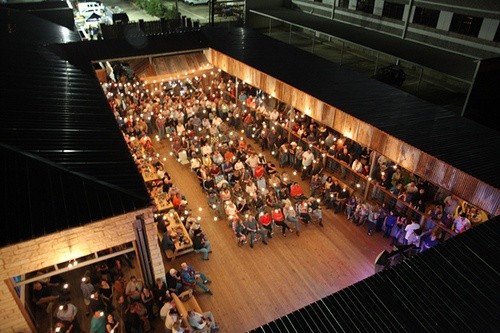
[208,251,212,253]
[207,290,212,295]
[269,235,273,238]
[284,234,287,238]
[203,258,209,260]
[319,223,323,227]
[250,244,253,248]
[203,280,212,284]
[296,231,299,236]
[263,241,267,245]
[289,230,293,233]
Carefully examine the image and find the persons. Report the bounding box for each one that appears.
[102,63,490,261]
[32,244,219,333]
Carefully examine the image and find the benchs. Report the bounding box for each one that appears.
[322,171,355,209]
[171,289,211,333]
[153,222,174,259]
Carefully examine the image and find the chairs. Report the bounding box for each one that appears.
[166,127,322,245]
[344,201,368,226]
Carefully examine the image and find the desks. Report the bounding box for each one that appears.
[130,140,193,261]
